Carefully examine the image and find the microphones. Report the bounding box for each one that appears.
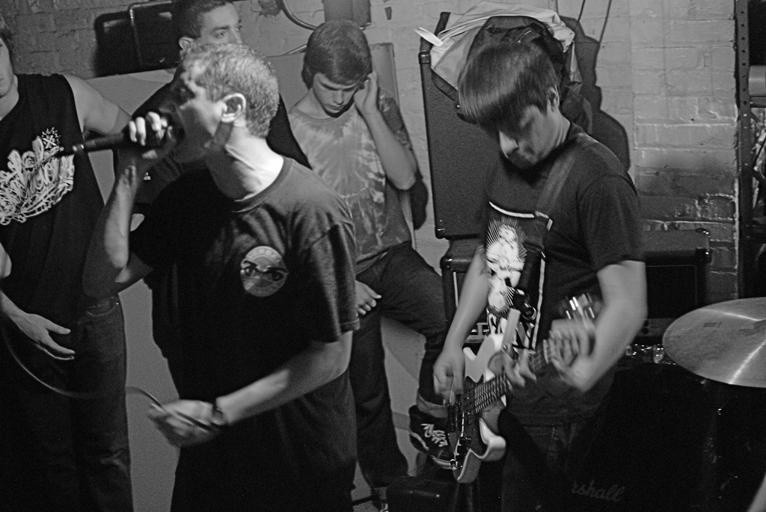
[62,121,186,158]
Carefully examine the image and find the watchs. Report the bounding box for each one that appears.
[210,395,230,435]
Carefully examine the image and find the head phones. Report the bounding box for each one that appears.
[301,55,371,90]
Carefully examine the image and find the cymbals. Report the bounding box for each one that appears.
[662,296,766,388]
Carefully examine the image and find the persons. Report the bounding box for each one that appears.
[287,18,455,511]
[431,42,650,510]
[0,18,133,511]
[81,42,358,511]
[112,0,314,292]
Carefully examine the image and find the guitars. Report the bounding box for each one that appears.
[448,294,597,484]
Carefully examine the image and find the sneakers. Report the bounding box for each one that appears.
[410,407,455,470]
